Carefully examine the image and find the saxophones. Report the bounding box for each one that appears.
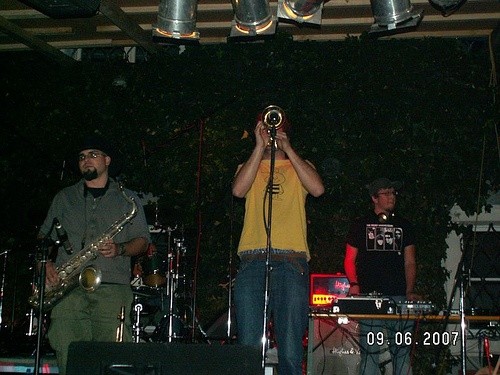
[27,180,137,309]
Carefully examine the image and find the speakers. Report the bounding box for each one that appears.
[65,342,266,375]
[307,317,394,375]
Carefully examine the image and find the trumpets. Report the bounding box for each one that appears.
[261,105,286,130]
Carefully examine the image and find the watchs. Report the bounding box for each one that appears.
[349,282,359,287]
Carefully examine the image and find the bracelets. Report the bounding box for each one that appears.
[119,243,127,255]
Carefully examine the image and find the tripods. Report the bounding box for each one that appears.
[141,231,213,343]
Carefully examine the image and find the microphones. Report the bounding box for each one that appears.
[55,220,74,255]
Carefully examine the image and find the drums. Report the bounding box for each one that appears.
[137,250,167,287]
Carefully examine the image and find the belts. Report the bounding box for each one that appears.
[241,254,306,272]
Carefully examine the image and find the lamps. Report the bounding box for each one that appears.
[150,0,467,46]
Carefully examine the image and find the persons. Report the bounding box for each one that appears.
[231,114,325,375]
[36,136,151,375]
[344,178,421,375]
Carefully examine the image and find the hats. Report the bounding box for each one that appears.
[257,112,290,132]
[368,177,401,195]
[76,136,112,159]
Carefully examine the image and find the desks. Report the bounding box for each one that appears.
[306,312,500,375]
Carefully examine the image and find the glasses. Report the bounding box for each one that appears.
[78,152,106,161]
[380,191,398,196]
